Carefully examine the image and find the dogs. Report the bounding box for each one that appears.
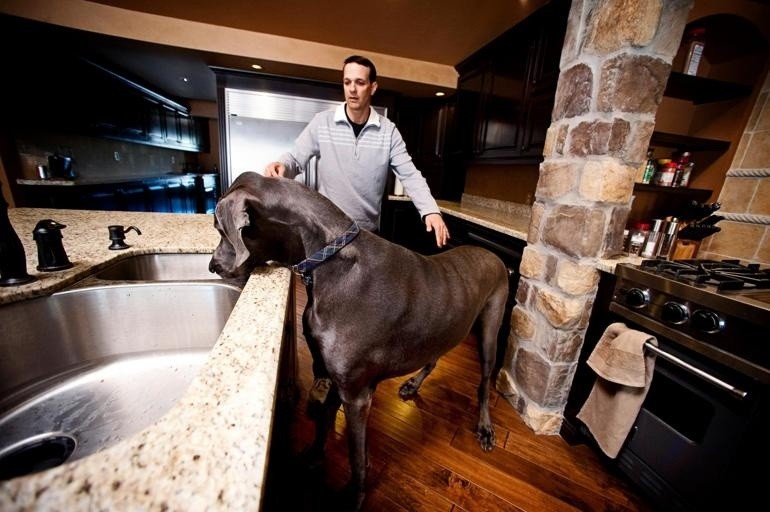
[208,170,510,512]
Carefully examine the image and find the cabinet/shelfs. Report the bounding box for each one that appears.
[73,59,211,156]
[10,173,221,218]
[383,201,525,359]
[633,60,749,201]
[440,23,568,172]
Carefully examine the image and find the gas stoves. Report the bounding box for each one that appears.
[603,258,770,381]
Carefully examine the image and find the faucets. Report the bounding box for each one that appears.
[1,178,38,285]
[108,225,142,250]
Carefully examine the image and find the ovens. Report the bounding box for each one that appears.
[582,322,770,512]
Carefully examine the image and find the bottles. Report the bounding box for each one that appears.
[644,148,694,188]
[619,216,678,262]
[34,164,48,180]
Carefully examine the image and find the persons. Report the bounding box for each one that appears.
[263,54,454,420]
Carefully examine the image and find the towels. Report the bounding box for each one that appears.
[575,317,662,462]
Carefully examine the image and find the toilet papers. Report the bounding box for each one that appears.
[394,177,403,196]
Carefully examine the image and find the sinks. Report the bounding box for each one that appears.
[95,248,248,282]
[0,283,243,483]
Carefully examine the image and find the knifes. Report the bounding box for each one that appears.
[678,200,724,241]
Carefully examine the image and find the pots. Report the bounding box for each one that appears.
[48,154,72,178]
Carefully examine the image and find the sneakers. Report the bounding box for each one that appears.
[309,376,333,406]
